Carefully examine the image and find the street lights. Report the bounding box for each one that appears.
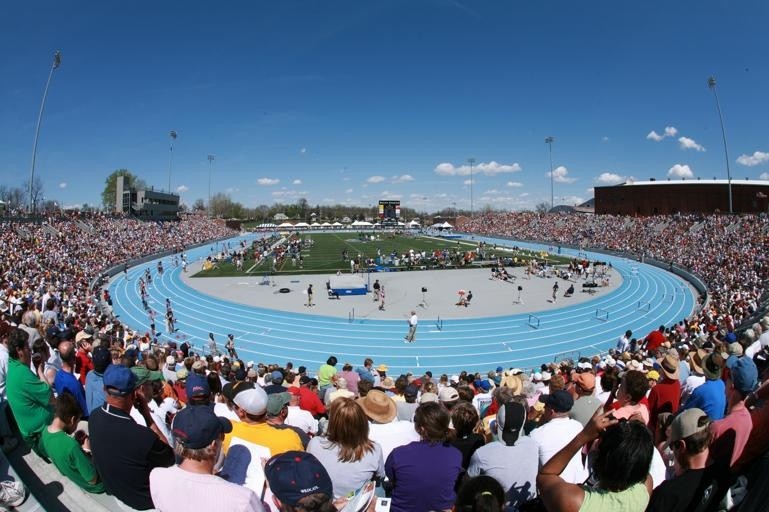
[27,51,62,215]
[707,75,734,213]
[467,156,475,218]
[207,152,215,219]
[168,130,178,194]
[545,136,555,213]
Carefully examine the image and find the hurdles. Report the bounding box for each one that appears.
[528,315,540,329]
[348,308,355,322]
[594,309,609,322]
[437,316,444,331]
[633,285,687,313]
[628,260,642,282]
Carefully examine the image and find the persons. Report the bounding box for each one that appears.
[0,196,769,512]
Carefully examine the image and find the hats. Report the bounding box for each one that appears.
[419,329,758,451]
[76,331,92,347]
[103,355,397,505]
